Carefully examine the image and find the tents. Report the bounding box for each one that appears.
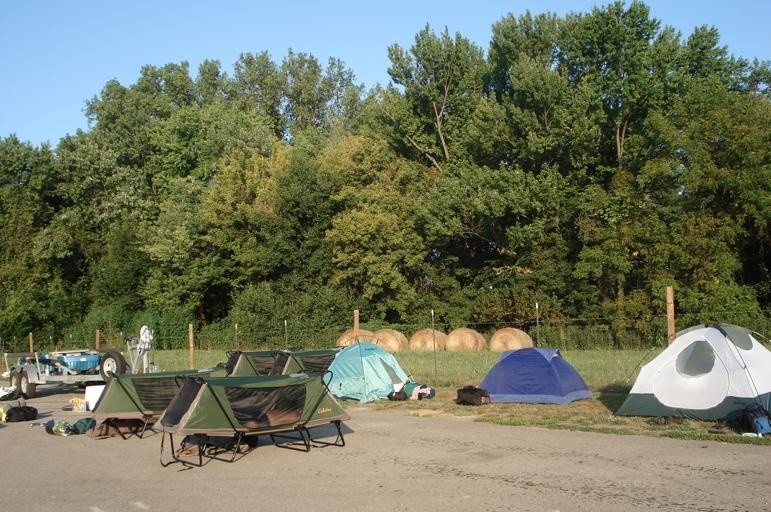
[151,374,351,437]
[478,347,593,406]
[91,367,228,412]
[614,321,771,423]
[224,351,278,376]
[324,341,410,403]
[268,349,340,375]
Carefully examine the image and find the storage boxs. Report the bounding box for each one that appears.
[33,352,56,376]
[57,353,102,374]
